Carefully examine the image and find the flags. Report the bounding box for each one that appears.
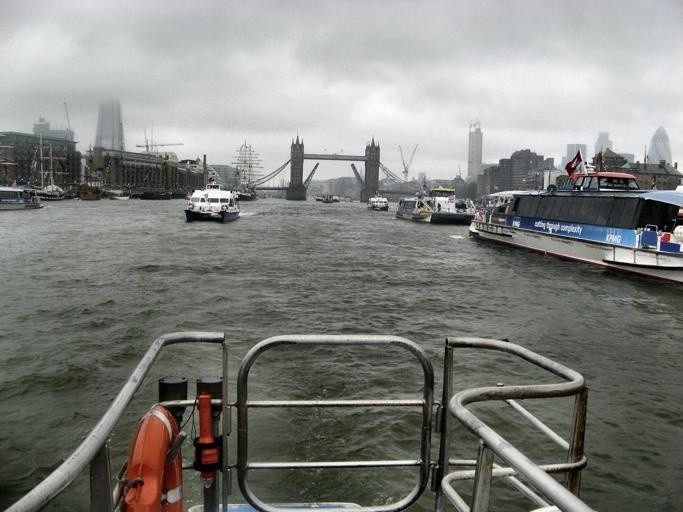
[563,148,582,177]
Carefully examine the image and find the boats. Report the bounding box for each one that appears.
[467,145,683,284]
[394,184,477,225]
[314,192,390,212]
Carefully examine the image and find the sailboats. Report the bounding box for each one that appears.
[0,130,265,212]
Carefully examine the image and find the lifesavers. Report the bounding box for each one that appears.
[123,403,183,511]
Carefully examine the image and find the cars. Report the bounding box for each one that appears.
[182,181,241,224]
